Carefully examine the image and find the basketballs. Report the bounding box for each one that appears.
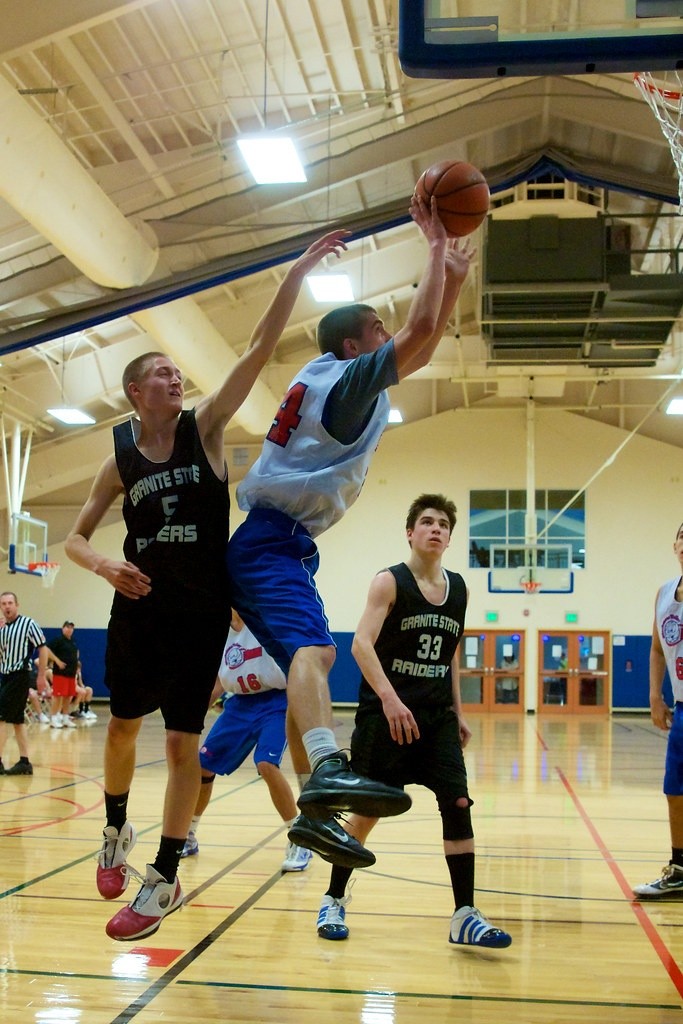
[414,160,490,239]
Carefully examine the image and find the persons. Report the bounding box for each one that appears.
[631,523,683,898]
[64,228,354,941]
[316,494,513,949]
[226,196,478,868]
[180,609,314,874]
[0,592,97,777]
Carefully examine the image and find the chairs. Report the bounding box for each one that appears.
[23,699,50,723]
[547,677,565,706]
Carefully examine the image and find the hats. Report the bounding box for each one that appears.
[63,620,74,627]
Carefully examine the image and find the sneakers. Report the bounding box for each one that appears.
[282,843,312,873]
[62,719,76,727]
[39,714,50,723]
[287,814,375,868]
[50,720,63,728]
[632,862,683,899]
[79,711,89,718]
[316,878,355,940]
[0,763,5,774]
[95,817,136,899]
[5,761,33,775]
[87,710,96,718]
[296,748,411,822]
[105,862,184,941]
[180,836,199,857]
[448,905,511,948]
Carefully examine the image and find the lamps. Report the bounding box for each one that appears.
[303,95,355,303]
[665,397,682,416]
[235,0,307,184]
[387,408,403,423]
[44,336,96,424]
